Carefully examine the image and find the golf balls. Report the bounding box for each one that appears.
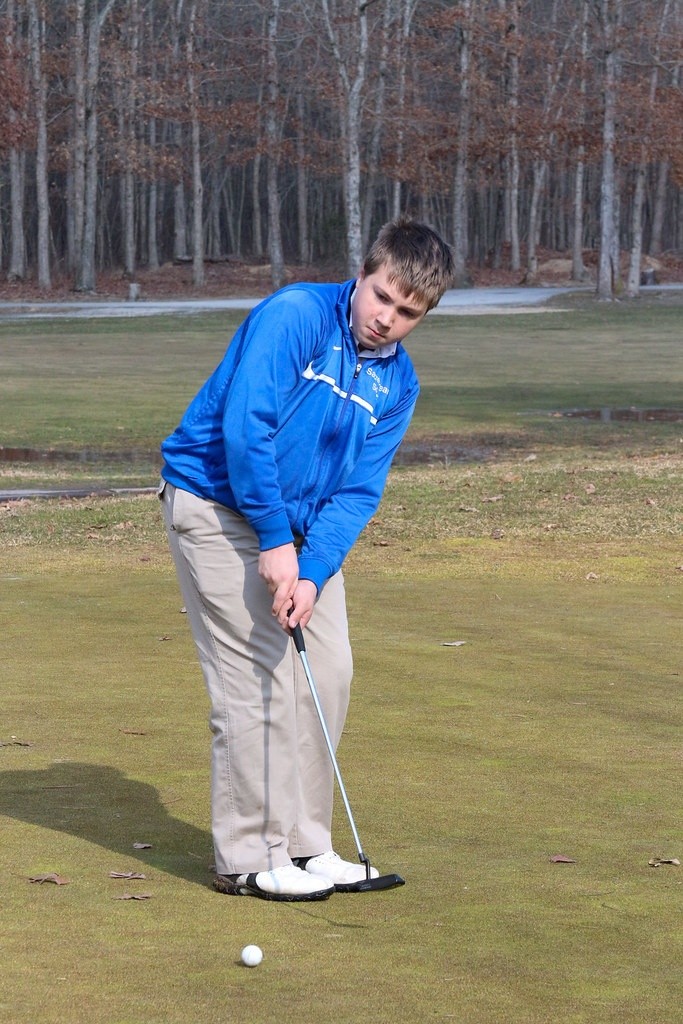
[241,943,264,967]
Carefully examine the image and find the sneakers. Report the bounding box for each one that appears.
[213,862,335,901]
[290,850,379,892]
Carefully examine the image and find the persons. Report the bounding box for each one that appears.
[161,210,456,903]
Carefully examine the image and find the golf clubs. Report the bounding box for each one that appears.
[287,602,406,894]
